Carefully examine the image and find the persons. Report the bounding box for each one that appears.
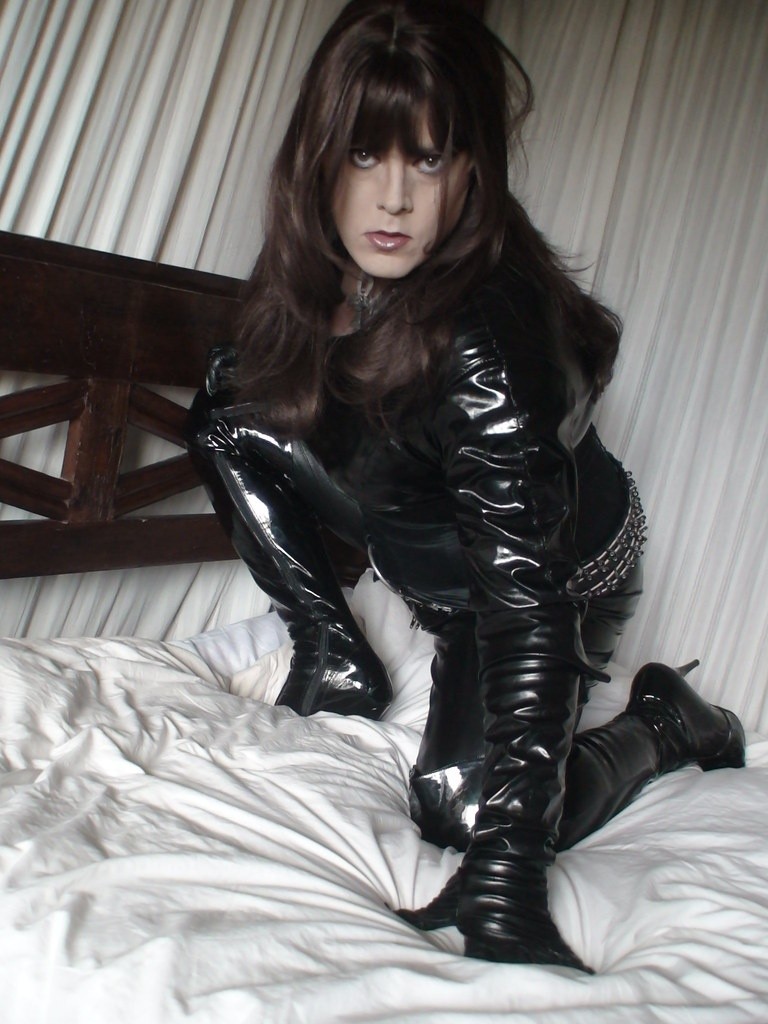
[186,3,746,978]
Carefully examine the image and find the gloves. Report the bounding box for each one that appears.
[205,351,236,397]
[397,854,597,975]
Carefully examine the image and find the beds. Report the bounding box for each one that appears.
[0,230,767,1023]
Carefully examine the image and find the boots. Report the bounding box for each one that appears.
[409,660,746,855]
[187,393,392,721]
